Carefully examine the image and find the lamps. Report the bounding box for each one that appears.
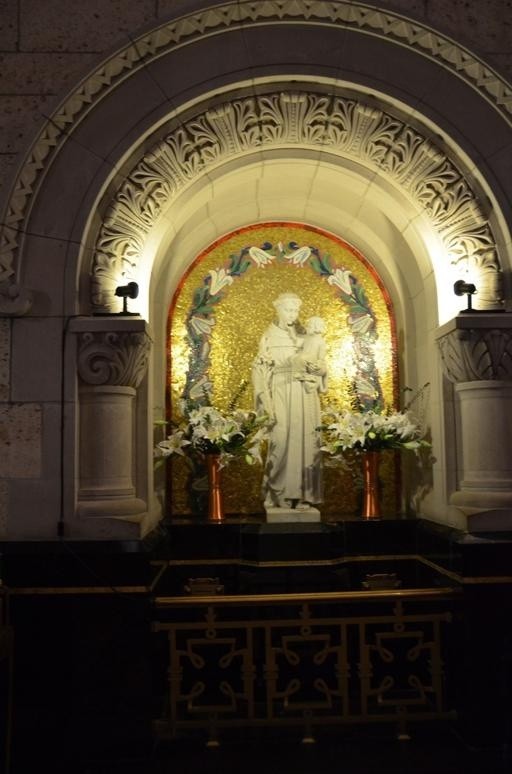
[114,281,140,316]
[453,280,477,313]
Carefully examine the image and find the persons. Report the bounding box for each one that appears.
[251,291,328,511]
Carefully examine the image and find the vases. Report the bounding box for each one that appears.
[362,451,383,520]
[205,452,227,521]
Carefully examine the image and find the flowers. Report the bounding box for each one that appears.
[317,375,436,465]
[151,405,269,471]
